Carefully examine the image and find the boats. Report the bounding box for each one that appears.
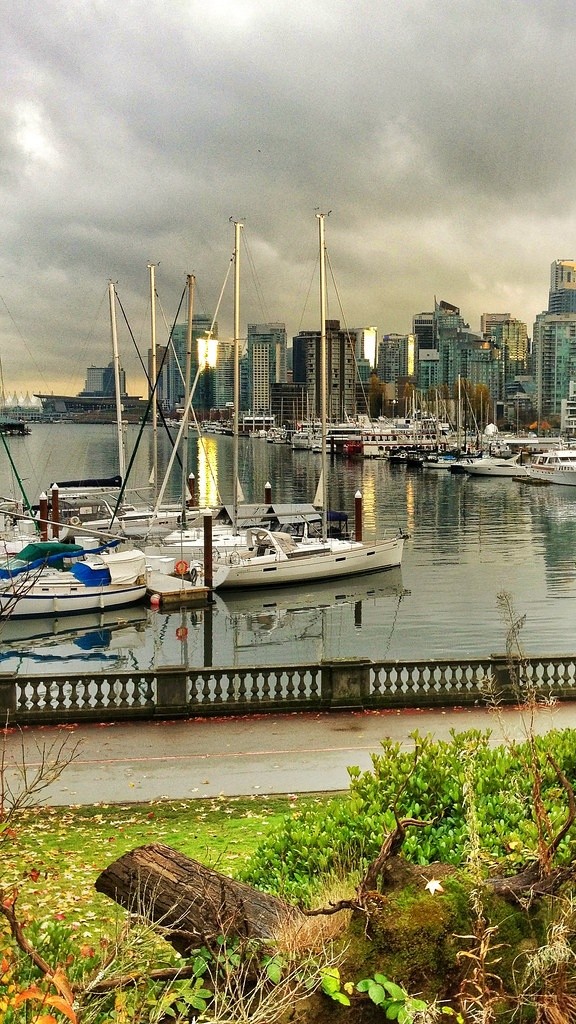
[0,605,156,666]
[213,564,411,695]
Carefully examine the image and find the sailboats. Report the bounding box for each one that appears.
[0,205,576,626]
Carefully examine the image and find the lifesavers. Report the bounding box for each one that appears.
[175,560,189,575]
[177,626,189,639]
[70,516,80,526]
